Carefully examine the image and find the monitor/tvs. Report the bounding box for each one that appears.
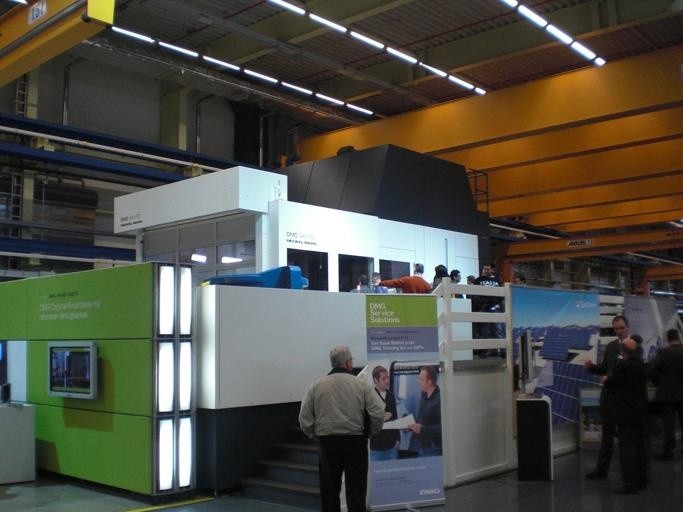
[46,341,99,403]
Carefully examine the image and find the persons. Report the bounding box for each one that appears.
[406,366,442,458]
[368,366,401,462]
[299,346,385,512]
[583,315,682,495]
[347,265,529,360]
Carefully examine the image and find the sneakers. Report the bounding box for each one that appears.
[585,468,606,479]
[614,477,647,494]
[653,453,673,460]
